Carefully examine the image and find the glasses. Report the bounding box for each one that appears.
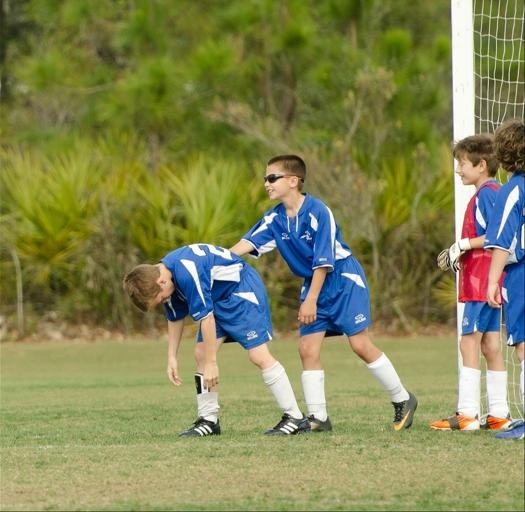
[264,173,304,183]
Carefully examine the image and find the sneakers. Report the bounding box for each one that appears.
[264,412,332,436]
[178,416,221,437]
[429,412,524,441]
[391,391,418,431]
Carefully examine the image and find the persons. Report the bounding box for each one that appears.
[122,243,313,438]
[486,116,525,440]
[226,154,417,431]
[429,135,512,431]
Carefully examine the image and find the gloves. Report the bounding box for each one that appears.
[437,237,472,272]
